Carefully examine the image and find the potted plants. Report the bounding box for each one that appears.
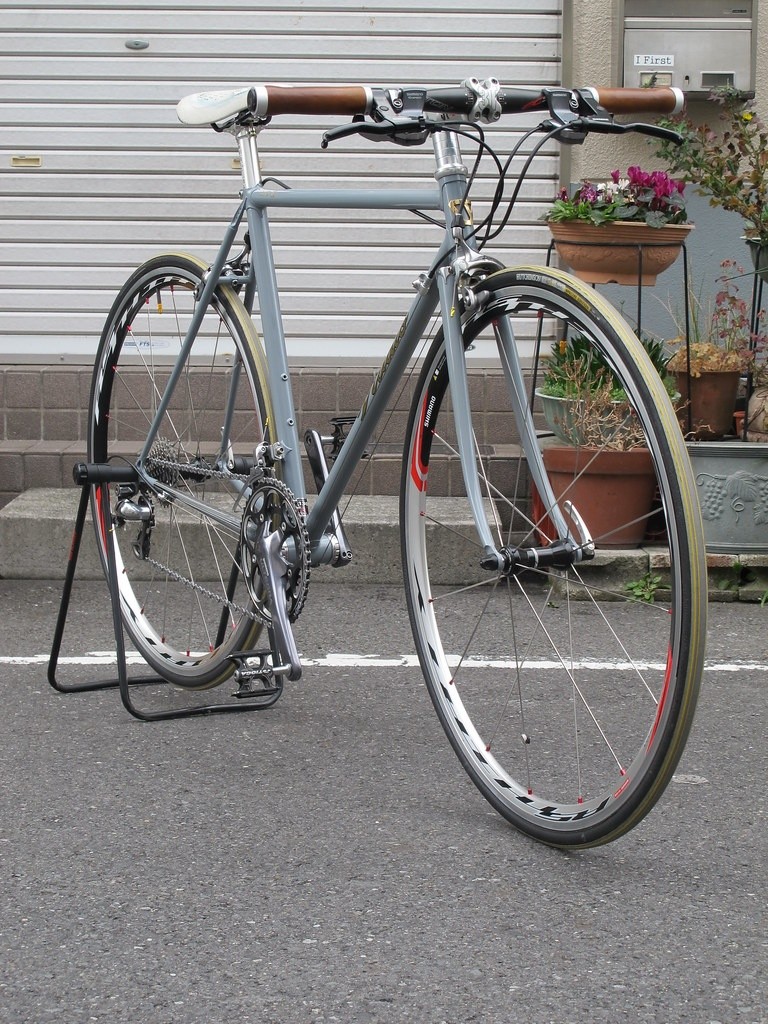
[529,366,694,548]
[664,340,745,442]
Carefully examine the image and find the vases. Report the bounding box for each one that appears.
[532,388,682,446]
[733,411,746,439]
[543,217,696,287]
[739,236,768,284]
[747,386,768,443]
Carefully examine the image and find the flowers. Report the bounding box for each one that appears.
[536,164,694,231]
[710,256,768,391]
[537,325,682,401]
[640,71,768,247]
